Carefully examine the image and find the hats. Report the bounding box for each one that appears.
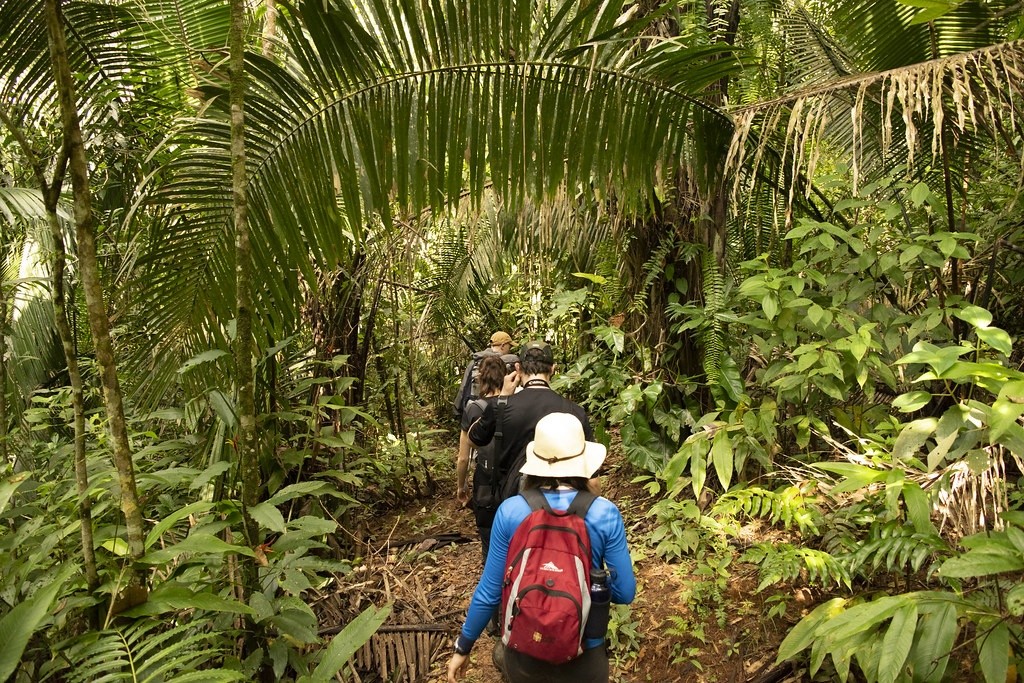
[520,340,554,364]
[490,331,519,347]
[519,412,607,478]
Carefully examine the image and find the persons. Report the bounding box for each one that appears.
[467,339,600,671]
[456,356,508,636]
[449,412,636,683]
[454,330,519,414]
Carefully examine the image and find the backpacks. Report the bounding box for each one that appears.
[500,486,617,665]
[474,485,500,527]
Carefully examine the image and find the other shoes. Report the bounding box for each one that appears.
[486,620,501,641]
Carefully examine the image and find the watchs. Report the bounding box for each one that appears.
[453,637,469,656]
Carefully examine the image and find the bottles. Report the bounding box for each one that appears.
[589,569,617,603]
